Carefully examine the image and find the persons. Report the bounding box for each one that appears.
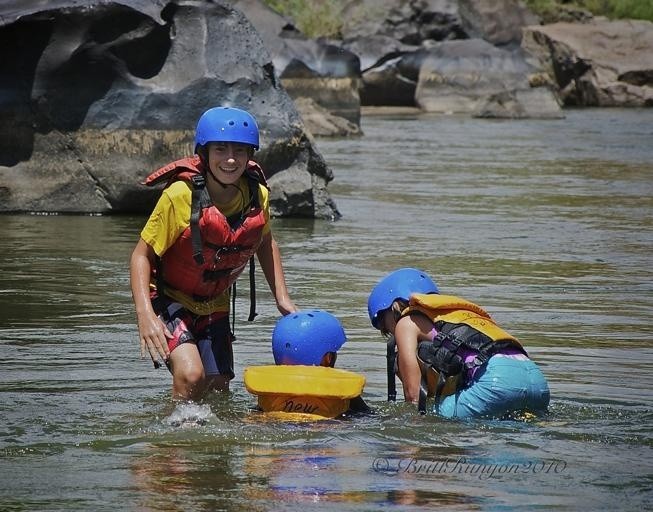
[243,306,371,423]
[366,265,551,424]
[126,105,302,418]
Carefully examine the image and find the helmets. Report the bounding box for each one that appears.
[194,107,260,156]
[272,309,347,367]
[368,268,440,331]
